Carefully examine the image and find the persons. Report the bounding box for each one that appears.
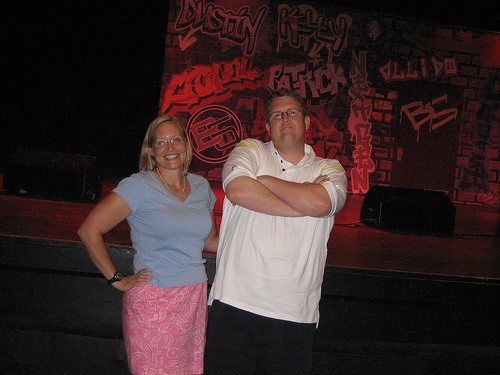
[203,89,348,375]
[77,114,219,375]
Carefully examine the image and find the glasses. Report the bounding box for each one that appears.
[268,110,305,120]
[151,138,186,146]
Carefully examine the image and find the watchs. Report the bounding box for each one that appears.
[108,271,124,287]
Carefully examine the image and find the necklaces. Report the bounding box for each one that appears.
[155,168,186,195]
